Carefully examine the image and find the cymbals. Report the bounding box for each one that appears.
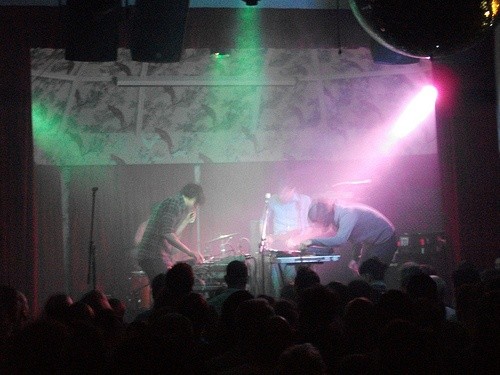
[204,231,239,245]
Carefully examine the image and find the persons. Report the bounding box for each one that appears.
[139,183,208,308]
[1,199,500,375]
[259,175,316,285]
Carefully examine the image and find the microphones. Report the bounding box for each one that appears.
[265,193,271,206]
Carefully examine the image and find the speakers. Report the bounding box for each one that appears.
[129,0,190,62]
[62,0,124,62]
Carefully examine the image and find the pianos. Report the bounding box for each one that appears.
[265,254,344,271]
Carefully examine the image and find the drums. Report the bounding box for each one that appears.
[118,270,151,311]
